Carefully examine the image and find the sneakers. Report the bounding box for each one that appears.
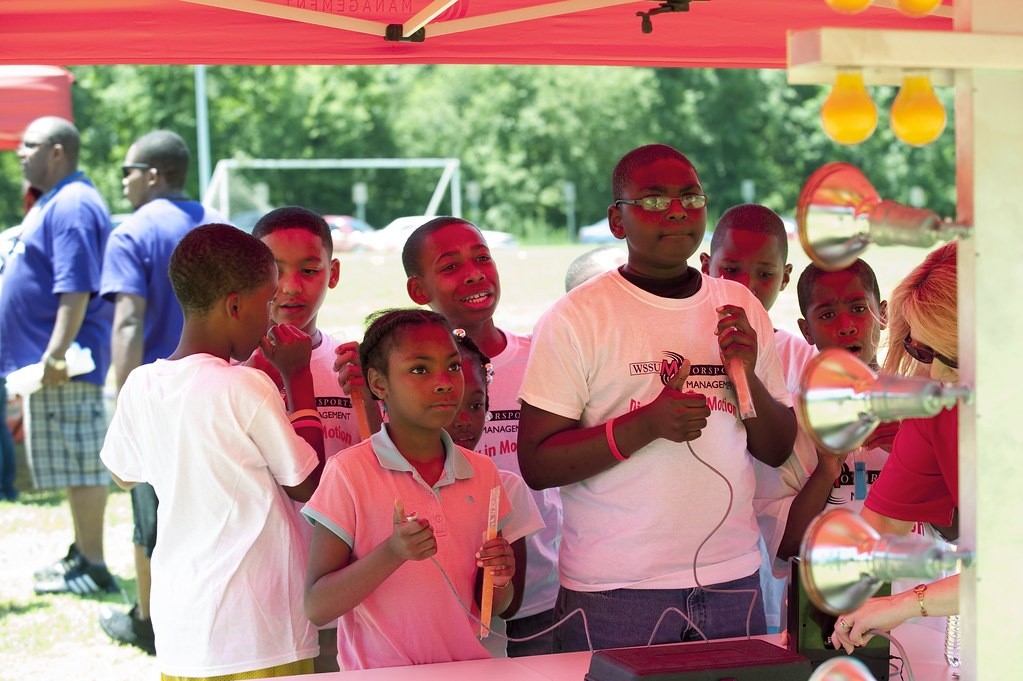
[35,561,119,595]
[34,544,87,579]
[101,603,156,656]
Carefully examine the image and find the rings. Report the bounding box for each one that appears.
[840,619,850,628]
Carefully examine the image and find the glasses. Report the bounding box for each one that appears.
[121,164,152,176]
[903,331,957,369]
[615,194,708,212]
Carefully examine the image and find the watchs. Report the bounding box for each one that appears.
[41,352,67,370]
[914,584,929,617]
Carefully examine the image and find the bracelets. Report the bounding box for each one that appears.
[493,581,510,589]
[291,419,323,431]
[606,418,630,462]
[288,408,321,423]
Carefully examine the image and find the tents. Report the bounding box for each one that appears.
[0,0,964,230]
[0,65,76,150]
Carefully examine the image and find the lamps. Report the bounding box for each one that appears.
[798,161,975,272]
[799,509,970,612]
[796,348,972,455]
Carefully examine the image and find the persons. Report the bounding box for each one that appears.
[98,130,239,651]
[1,185,44,501]
[443,329,546,660]
[698,203,820,634]
[229,207,384,673]
[514,145,803,649]
[298,308,519,673]
[400,215,566,656]
[99,224,322,681]
[0,116,123,595]
[753,252,906,584]
[826,241,960,655]
